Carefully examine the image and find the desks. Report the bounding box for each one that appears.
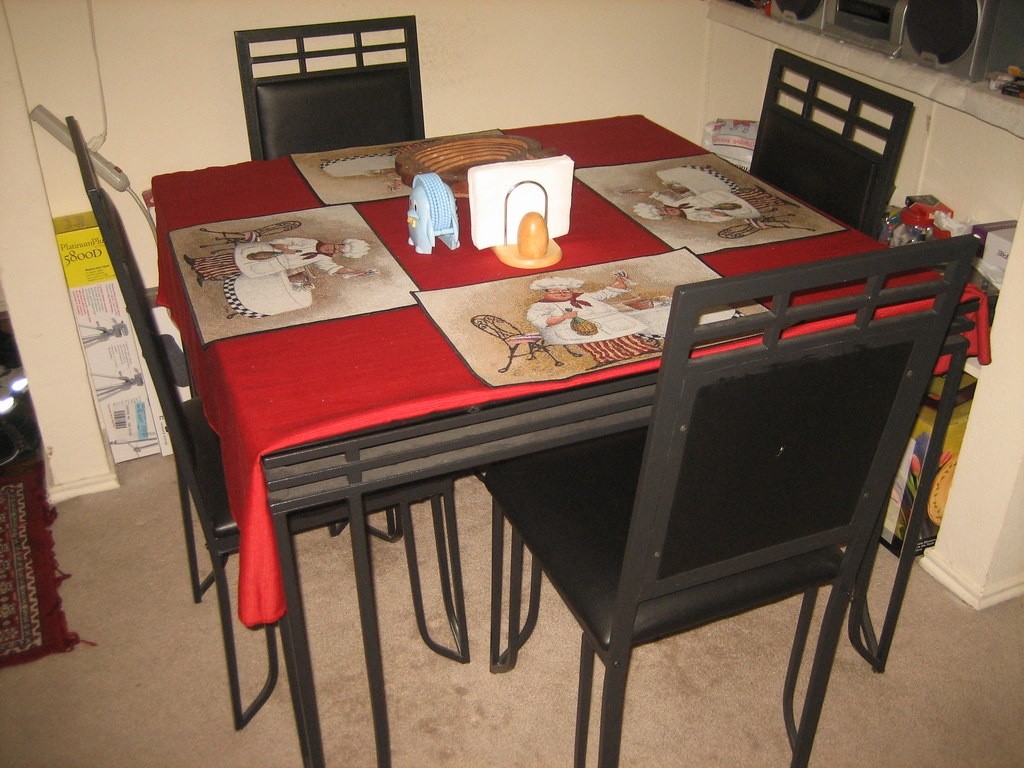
[150,114,990,768]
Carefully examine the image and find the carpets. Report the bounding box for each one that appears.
[0,461,98,671]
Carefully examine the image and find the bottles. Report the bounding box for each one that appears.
[889,207,935,248]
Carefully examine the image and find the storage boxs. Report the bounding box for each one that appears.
[905,194,954,225]
[52,211,193,465]
[971,219,1017,271]
[877,370,979,559]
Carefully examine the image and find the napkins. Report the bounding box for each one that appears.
[467,155,576,250]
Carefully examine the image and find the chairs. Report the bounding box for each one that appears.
[234,14,429,542]
[745,49,917,240]
[473,234,980,768]
[66,115,471,730]
